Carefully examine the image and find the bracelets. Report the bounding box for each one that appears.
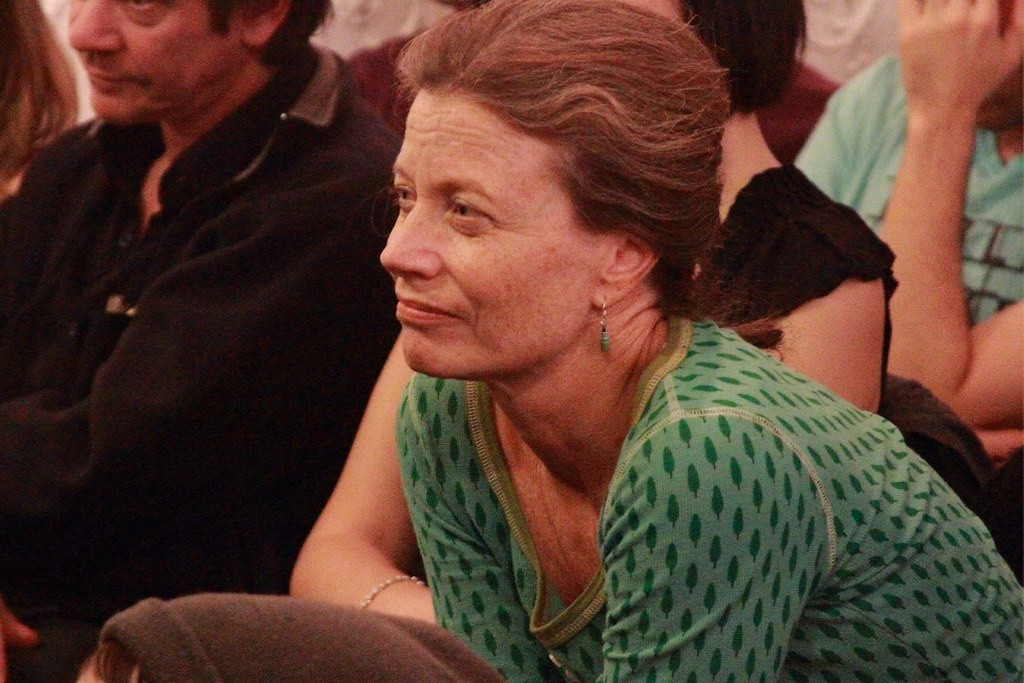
[360,575,425,609]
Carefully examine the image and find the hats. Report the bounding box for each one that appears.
[98,592,510,683]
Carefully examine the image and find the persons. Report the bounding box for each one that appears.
[629,0,901,413]
[0,0,78,182]
[0,0,403,683]
[793,0,1024,468]
[290,0,1024,683]
[77,592,504,683]
